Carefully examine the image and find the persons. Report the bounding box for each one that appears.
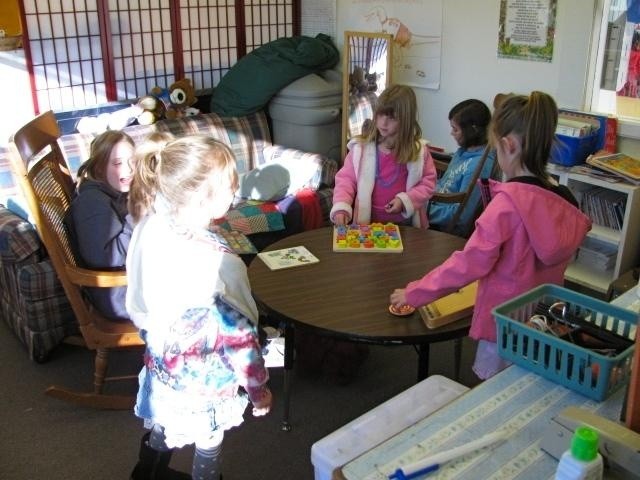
[64,130,142,325]
[421,95,502,239]
[390,87,596,388]
[122,132,275,480]
[327,83,438,235]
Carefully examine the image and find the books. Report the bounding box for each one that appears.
[555,114,640,279]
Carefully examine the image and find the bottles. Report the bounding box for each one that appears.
[555,427,604,479]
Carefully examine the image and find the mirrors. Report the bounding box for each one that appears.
[341,31,392,168]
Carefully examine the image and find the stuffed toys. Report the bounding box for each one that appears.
[137,73,198,134]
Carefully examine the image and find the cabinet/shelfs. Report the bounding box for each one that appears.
[541,167,640,296]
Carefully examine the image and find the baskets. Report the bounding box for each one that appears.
[490,283,640,404]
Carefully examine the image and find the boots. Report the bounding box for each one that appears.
[124,430,194,480]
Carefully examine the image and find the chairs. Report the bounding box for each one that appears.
[8,109,249,409]
[428,90,515,235]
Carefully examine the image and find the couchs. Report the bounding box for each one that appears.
[0,98,342,365]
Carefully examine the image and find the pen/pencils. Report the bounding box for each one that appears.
[385,428,510,480]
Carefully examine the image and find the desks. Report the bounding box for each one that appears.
[249,225,640,479]
